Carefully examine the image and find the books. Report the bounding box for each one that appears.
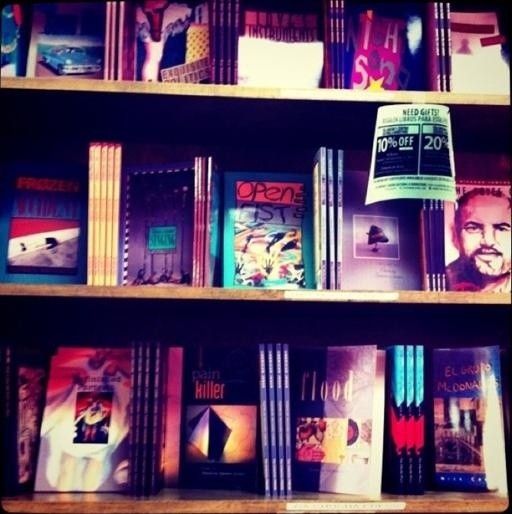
[2,340,506,498]
[0,1,511,97]
[0,144,512,293]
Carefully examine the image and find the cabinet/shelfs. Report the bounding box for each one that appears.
[0,76,512,514]
